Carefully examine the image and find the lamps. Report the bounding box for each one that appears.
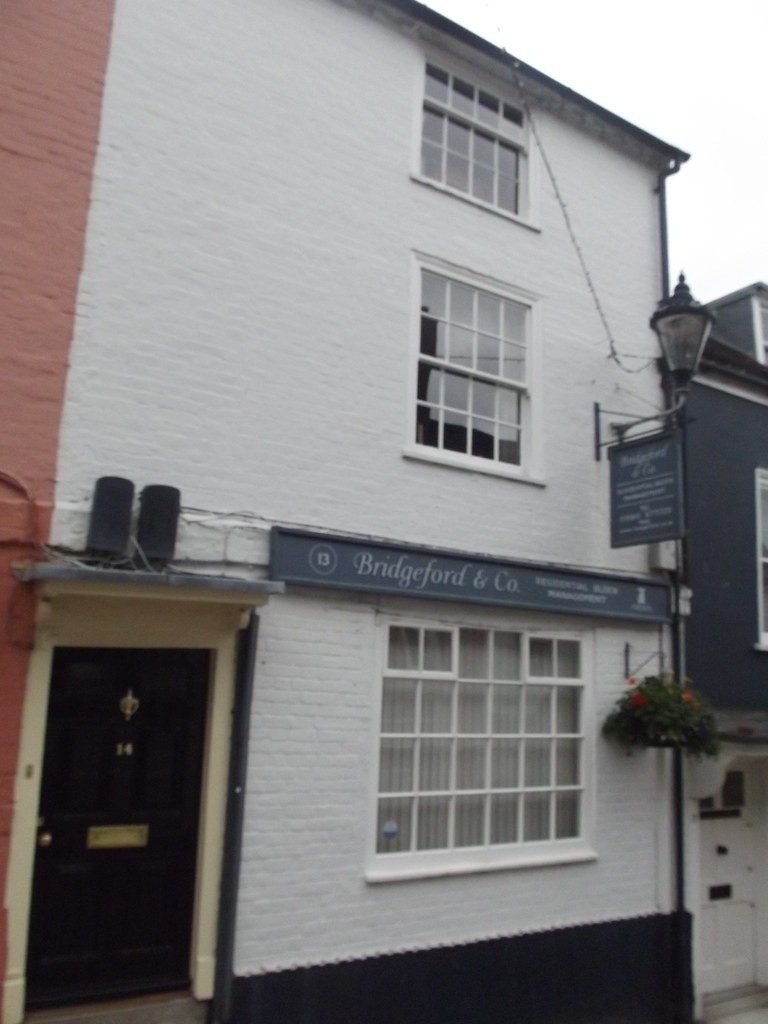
[593,269,717,460]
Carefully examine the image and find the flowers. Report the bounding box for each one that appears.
[608,672,720,765]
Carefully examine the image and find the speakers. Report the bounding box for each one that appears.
[137,484,180,562]
[85,476,133,558]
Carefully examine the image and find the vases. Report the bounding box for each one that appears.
[647,721,685,746]
